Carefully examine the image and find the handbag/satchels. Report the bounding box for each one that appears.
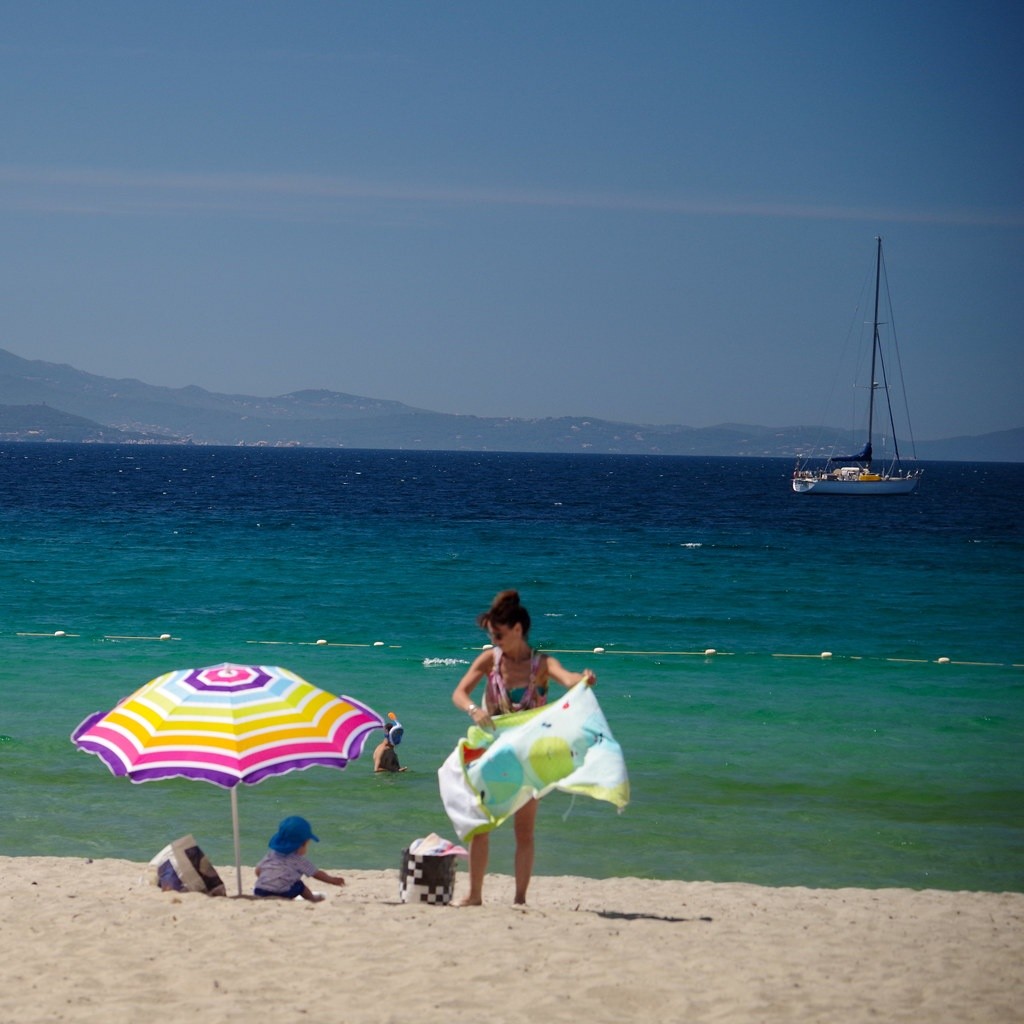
[148,835,226,897]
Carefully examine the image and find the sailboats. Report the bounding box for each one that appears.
[789,234,924,495]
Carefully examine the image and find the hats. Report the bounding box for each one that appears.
[267,816,319,853]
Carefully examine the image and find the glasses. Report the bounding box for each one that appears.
[487,629,510,640]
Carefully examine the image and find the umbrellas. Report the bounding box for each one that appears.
[71,662,384,898]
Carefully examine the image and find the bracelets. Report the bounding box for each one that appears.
[467,705,478,716]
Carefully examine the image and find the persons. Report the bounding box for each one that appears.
[373,723,407,772]
[254,815,344,902]
[453,591,597,908]
